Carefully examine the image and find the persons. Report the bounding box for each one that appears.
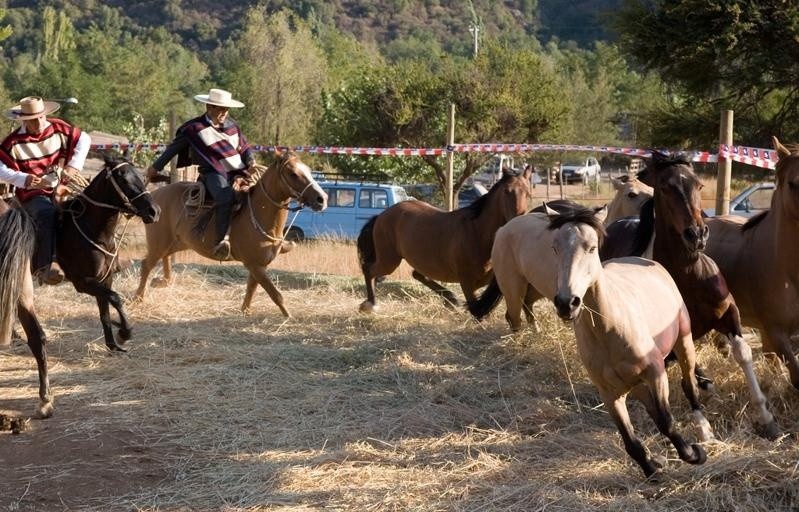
[0,95,92,286]
[144,88,297,262]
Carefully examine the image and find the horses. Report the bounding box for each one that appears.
[355,162,536,325]
[115,142,329,317]
[492,172,664,349]
[0,143,166,419]
[543,134,798,476]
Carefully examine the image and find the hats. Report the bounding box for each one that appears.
[3,96,61,121]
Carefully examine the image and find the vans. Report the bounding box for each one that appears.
[284,170,409,242]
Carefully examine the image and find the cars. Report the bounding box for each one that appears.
[555,156,602,186]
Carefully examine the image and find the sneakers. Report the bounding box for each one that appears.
[34,269,63,285]
[281,239,296,253]
[209,240,231,260]
[194,88,244,108]
